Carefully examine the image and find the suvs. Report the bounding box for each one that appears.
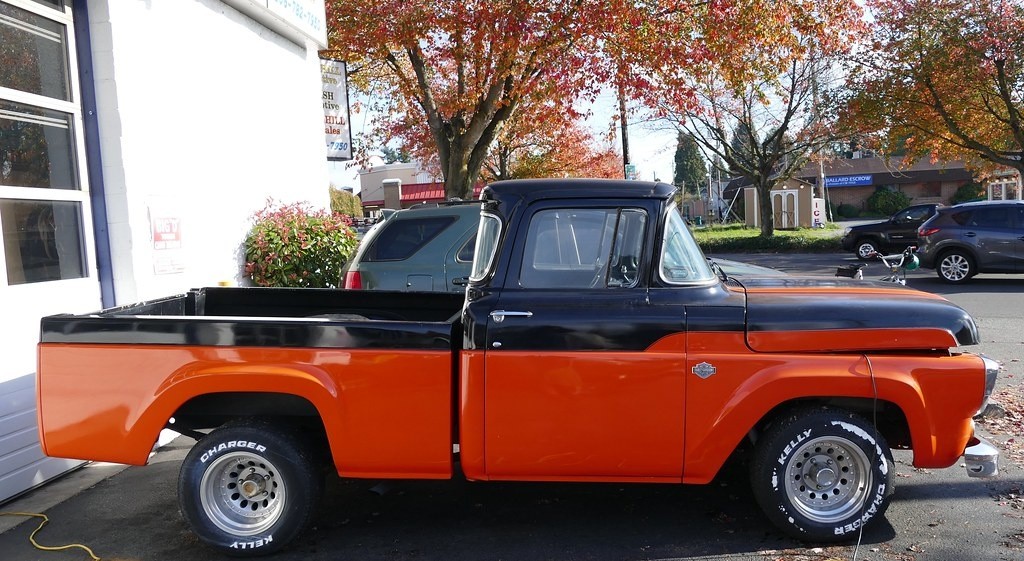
[336,195,789,289]
[915,199,1024,285]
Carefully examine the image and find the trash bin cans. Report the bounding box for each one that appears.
[695,216,701,225]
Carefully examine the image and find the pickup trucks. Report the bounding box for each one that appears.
[31,177,1010,560]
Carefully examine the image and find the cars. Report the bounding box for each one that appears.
[839,202,945,263]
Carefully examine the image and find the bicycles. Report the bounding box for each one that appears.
[834,244,917,286]
